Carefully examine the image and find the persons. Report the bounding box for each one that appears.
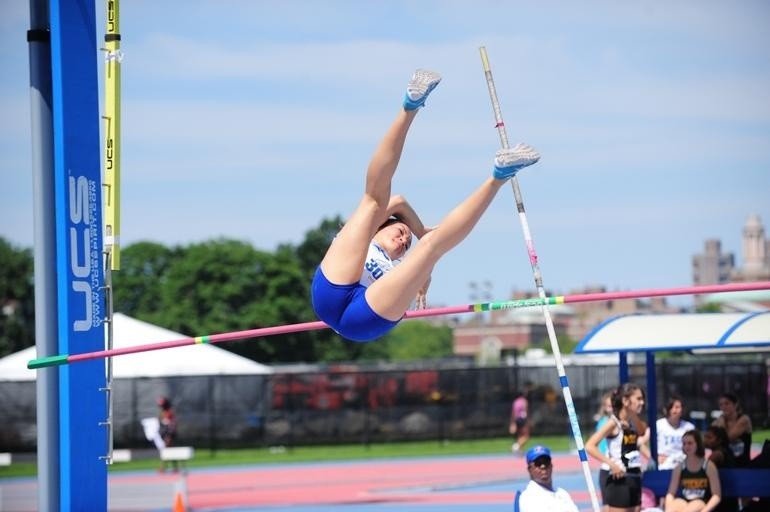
[506,381,770,512]
[310,70,541,343]
[155,397,178,475]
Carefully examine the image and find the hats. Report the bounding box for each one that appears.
[526,445,551,462]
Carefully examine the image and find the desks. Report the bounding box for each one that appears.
[637,464,770,512]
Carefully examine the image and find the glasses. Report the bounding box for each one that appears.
[528,458,551,466]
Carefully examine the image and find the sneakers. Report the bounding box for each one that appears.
[492,141,540,179]
[403,69,442,112]
